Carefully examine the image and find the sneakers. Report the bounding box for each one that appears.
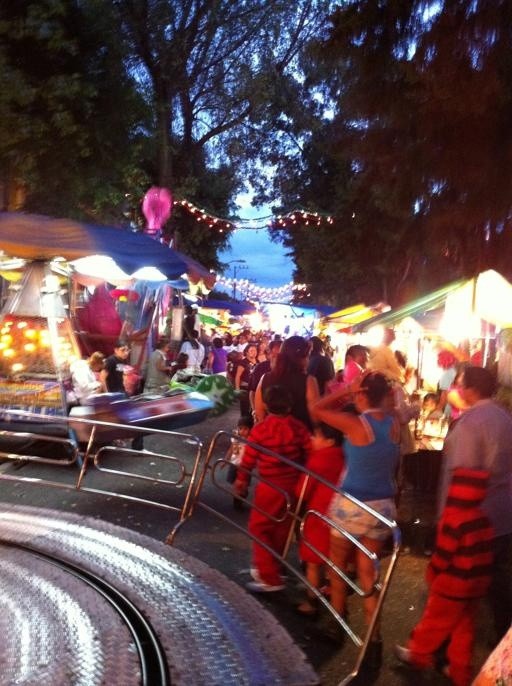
[246,567,450,680]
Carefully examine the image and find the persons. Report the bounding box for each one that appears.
[436,366,512,643]
[394,466,494,686]
[294,422,346,617]
[80,327,511,509]
[233,373,311,593]
[311,367,400,644]
